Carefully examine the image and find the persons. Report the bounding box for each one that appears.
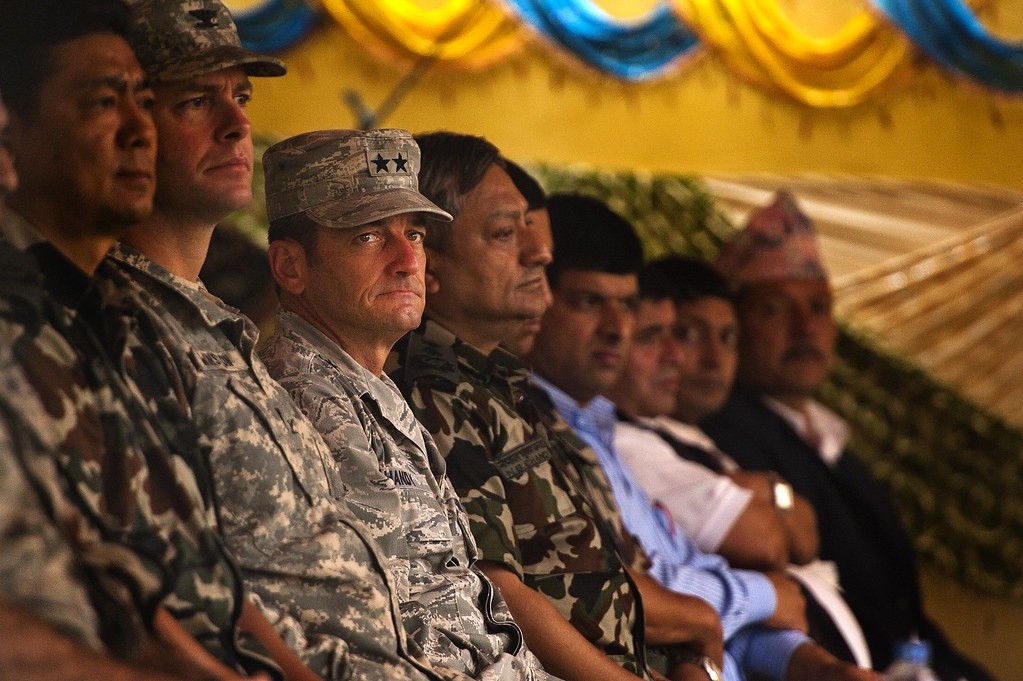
[0,0,325,681]
[130,0,473,681]
[384,131,724,681]
[696,240,995,681]
[608,257,889,681]
[256,131,548,681]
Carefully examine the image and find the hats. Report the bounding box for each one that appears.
[262,129,454,229]
[126,0,287,83]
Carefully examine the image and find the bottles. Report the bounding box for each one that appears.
[888,641,937,681]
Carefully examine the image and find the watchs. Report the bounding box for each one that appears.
[766,469,793,511]
[681,654,723,681]
